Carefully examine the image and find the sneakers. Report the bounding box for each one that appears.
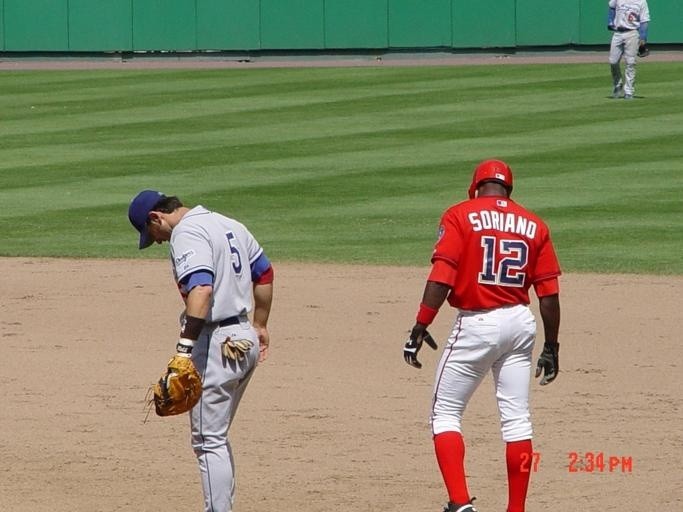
[613,85,622,98]
[442,497,476,512]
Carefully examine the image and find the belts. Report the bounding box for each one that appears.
[217,316,240,328]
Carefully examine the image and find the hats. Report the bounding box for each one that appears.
[128,190,165,249]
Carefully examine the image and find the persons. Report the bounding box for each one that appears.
[128,190,273,512]
[403,160,561,512]
[608,0,650,101]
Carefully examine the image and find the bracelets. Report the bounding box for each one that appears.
[176,338,198,358]
[416,303,438,325]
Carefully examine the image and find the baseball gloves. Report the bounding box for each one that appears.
[153,355,203,418]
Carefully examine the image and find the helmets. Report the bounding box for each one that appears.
[469,159,513,198]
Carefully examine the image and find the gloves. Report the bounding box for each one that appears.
[404,328,438,369]
[221,335,254,363]
[535,341,559,386]
